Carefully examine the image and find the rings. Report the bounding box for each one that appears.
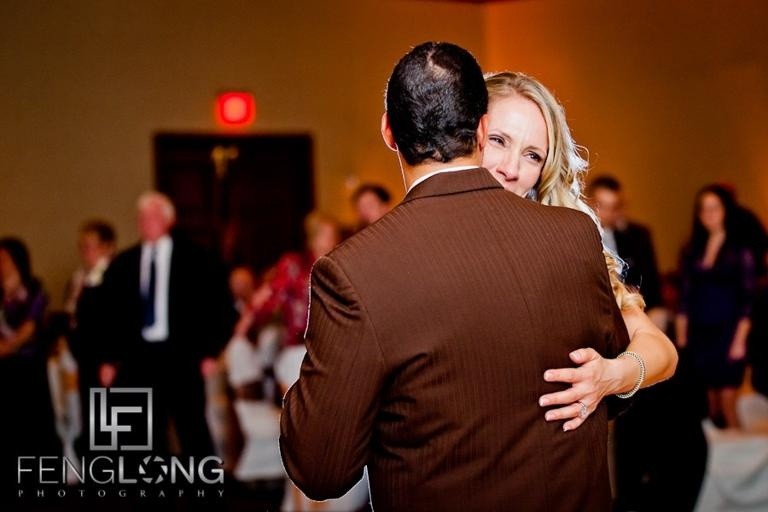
[577,399,588,418]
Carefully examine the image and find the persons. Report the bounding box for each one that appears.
[588,177,768,512]
[280,41,628,511]
[483,70,679,510]
[0,186,392,512]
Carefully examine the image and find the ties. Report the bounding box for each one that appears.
[143,245,156,330]
